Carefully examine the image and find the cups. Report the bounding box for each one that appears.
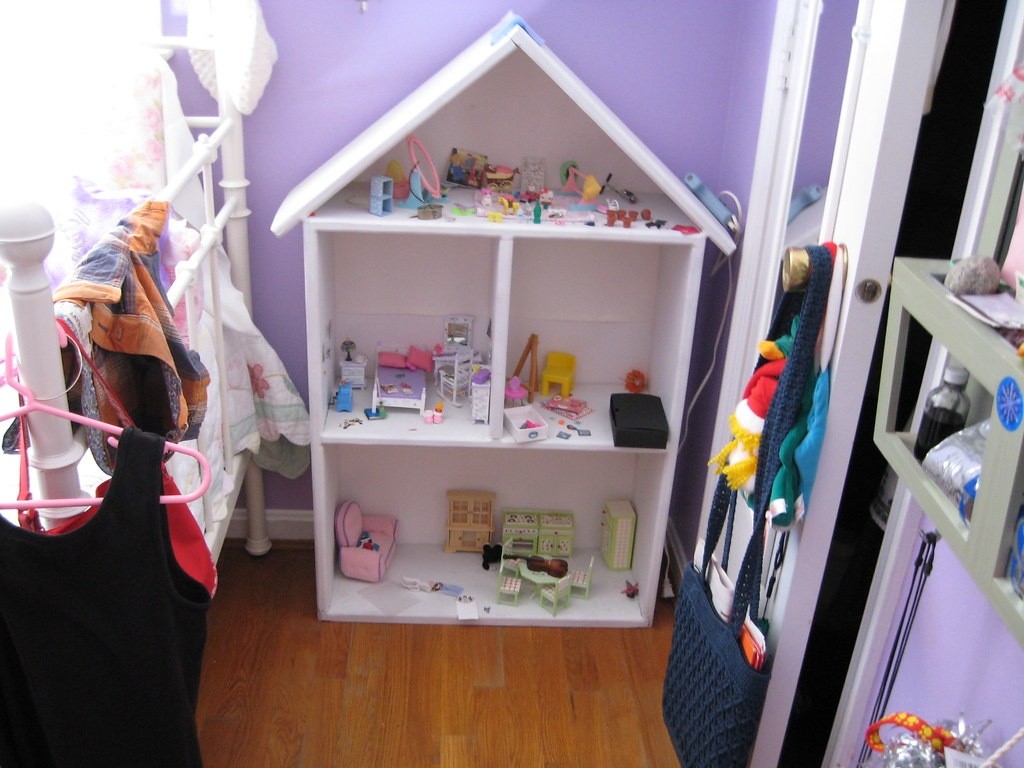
[623,218,631,228]
[607,217,616,226]
[641,209,650,219]
[607,210,615,218]
[629,211,637,221]
[617,210,626,220]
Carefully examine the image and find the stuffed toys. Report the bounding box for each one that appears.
[701,313,806,530]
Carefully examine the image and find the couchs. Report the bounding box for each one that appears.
[334,499,400,583]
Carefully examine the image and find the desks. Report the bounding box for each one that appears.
[433,352,481,387]
[518,555,559,600]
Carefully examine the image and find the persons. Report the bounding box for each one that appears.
[508,515,534,524]
[379,400,386,418]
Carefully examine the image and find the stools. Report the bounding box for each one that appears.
[504,386,526,407]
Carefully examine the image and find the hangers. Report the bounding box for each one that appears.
[0,320,213,510]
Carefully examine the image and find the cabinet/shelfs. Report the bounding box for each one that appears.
[471,364,490,425]
[444,489,497,553]
[270,11,738,629]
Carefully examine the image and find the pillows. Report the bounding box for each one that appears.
[404,346,433,374]
[377,352,406,369]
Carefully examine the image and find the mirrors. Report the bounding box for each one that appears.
[443,314,474,348]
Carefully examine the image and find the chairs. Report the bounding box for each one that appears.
[541,351,577,397]
[499,535,521,578]
[435,349,474,407]
[571,556,595,601]
[540,574,574,618]
[494,561,522,607]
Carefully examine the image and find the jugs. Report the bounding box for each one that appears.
[607,199,619,210]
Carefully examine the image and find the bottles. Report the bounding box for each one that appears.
[534,199,541,224]
[914,366,971,461]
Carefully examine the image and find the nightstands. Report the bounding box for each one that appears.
[339,356,368,391]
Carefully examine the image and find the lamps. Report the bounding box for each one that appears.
[340,336,357,361]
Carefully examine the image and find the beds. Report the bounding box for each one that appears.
[371,340,434,416]
[0,0,310,603]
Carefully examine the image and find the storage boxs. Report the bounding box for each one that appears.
[609,393,669,449]
[503,406,548,443]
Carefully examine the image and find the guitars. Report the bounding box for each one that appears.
[502,551,569,577]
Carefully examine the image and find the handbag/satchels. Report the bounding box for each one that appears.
[662,562,774,768]
